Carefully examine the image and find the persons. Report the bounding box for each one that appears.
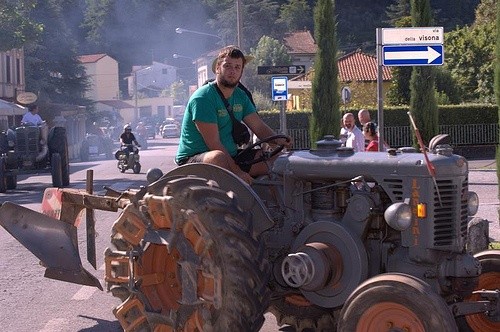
[119,124,141,158]
[136,122,146,135]
[175,47,292,213]
[20,102,43,124]
[361,122,388,152]
[358,109,379,135]
[86,122,104,140]
[340,112,364,152]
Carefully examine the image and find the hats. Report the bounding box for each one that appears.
[212,45,254,76]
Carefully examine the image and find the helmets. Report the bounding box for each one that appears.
[123,125,132,135]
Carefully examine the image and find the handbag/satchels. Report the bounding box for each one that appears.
[205,83,250,145]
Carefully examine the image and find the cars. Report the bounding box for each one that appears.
[160,117,181,138]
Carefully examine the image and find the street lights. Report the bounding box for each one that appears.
[135,66,151,118]
[172,52,193,60]
[175,27,227,47]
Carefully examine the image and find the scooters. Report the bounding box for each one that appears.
[117,144,141,174]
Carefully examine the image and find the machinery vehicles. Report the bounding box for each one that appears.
[104,132,500,332]
[0,122,70,192]
[79,119,156,162]
